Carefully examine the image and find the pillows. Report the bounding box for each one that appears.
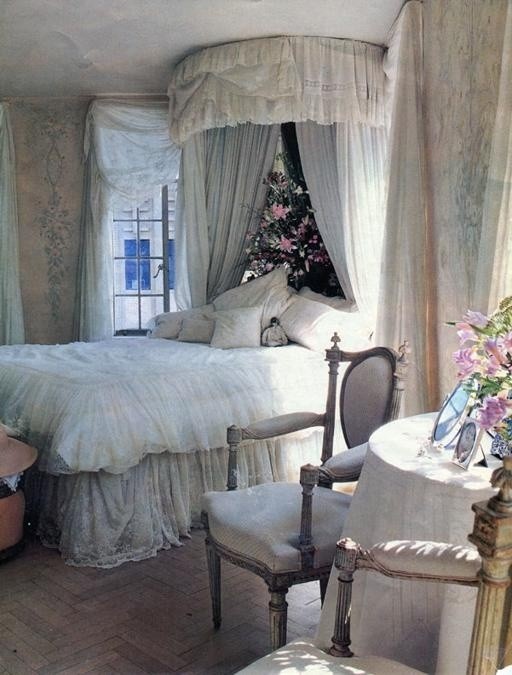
[178,319,213,343]
[212,264,287,313]
[299,286,345,309]
[146,305,215,338]
[278,295,357,353]
[211,306,262,348]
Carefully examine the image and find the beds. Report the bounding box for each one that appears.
[0,335,375,571]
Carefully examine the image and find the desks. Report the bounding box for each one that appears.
[313,411,501,674]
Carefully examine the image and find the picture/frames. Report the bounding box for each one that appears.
[452,416,488,471]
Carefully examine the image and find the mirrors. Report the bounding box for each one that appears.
[431,377,478,452]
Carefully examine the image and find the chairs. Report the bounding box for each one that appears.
[231,537,483,674]
[200,334,410,651]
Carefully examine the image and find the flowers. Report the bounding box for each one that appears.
[244,172,330,288]
[445,294,512,457]
